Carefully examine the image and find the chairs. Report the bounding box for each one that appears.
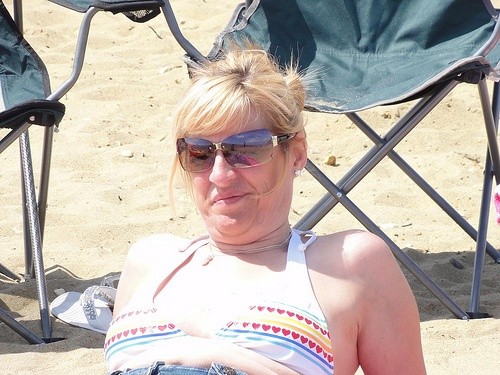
[187,0,500,320]
[1,1,164,346]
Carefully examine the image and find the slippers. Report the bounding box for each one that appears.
[49,285,121,336]
[96,274,121,289]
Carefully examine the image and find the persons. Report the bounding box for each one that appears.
[188,144,201,155]
[103,34,427,375]
[243,141,246,148]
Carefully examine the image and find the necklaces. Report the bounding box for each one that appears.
[200,228,292,267]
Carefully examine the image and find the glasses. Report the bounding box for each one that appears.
[171,127,299,173]
